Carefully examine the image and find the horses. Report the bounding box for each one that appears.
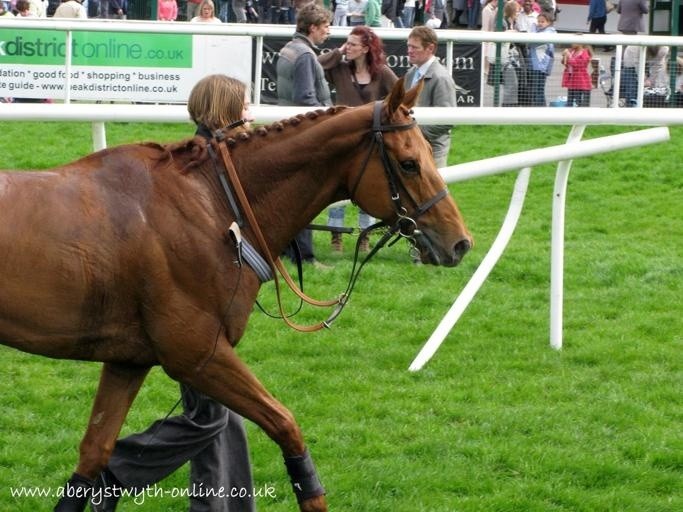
[0,71,474,511]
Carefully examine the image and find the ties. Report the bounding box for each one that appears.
[410,69,420,91]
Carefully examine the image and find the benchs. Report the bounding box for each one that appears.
[599,56,682,108]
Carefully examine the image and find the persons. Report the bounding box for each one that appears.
[403,25,456,168]
[88,75,256,512]
[274,1,341,271]
[0,1,682,106]
[318,26,398,250]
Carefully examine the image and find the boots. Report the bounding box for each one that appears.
[360,234,369,252]
[331,231,342,252]
